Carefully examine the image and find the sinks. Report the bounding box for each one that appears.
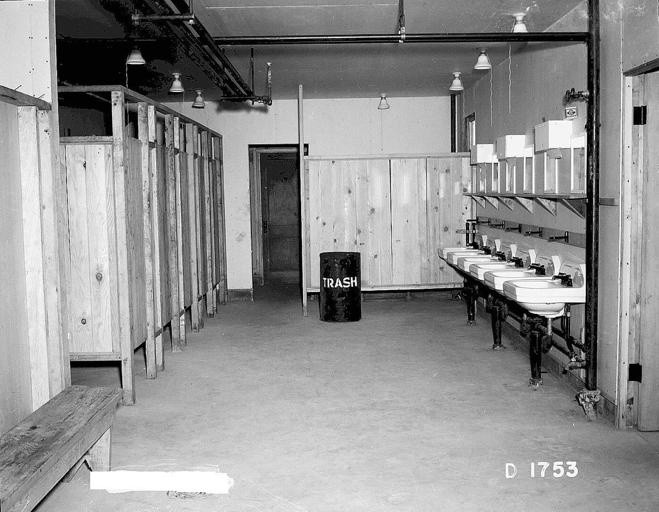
[502,278,586,312]
[457,257,507,273]
[437,247,481,260]
[468,263,527,281]
[483,270,552,291]
[446,252,492,265]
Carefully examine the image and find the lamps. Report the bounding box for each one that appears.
[126,44,206,108]
[449,11,527,92]
[377,93,390,110]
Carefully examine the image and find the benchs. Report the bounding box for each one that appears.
[0,383,122,512]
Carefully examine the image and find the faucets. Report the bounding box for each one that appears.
[479,246,492,255]
[510,257,524,267]
[527,263,546,276]
[466,241,479,249]
[553,272,573,287]
[494,251,506,261]
